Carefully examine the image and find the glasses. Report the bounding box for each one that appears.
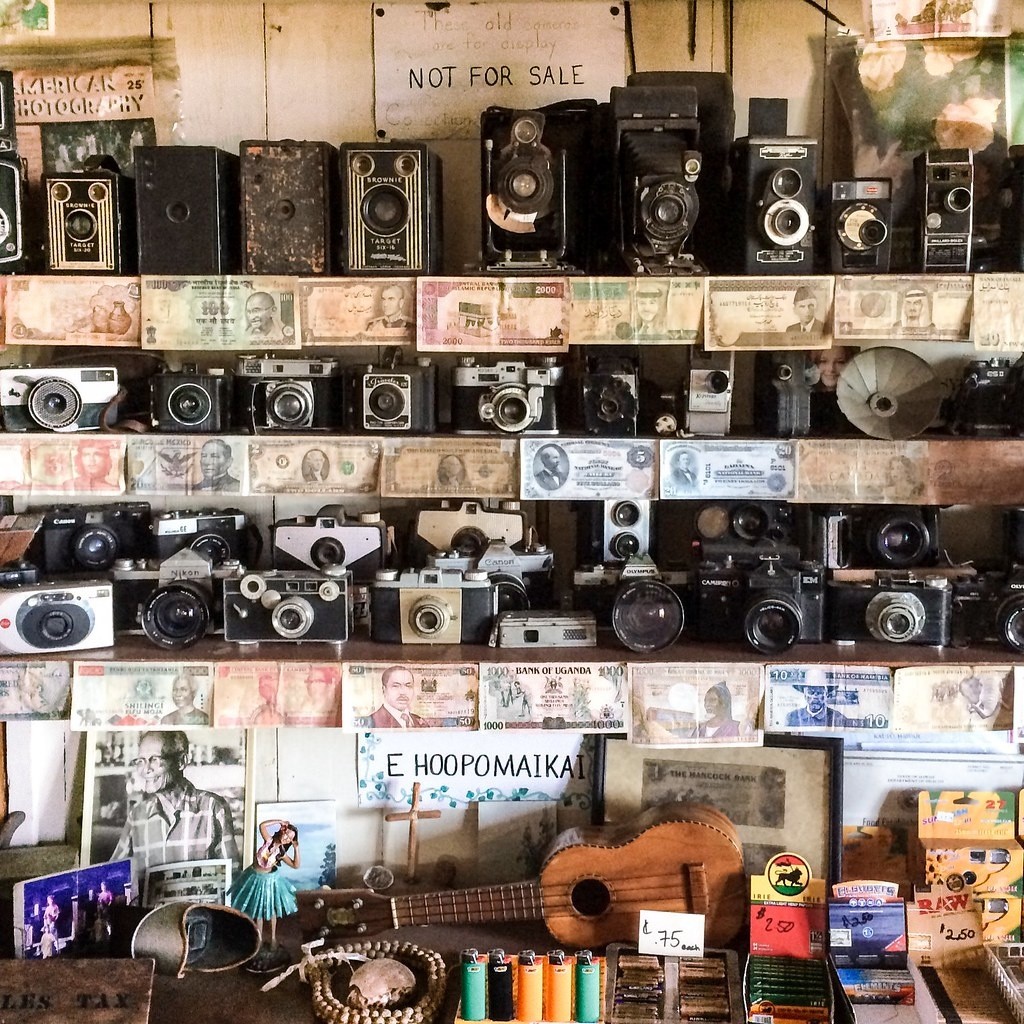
[134,752,181,766]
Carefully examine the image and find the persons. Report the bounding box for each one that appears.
[108,732,240,889]
[225,818,301,950]
[29,880,113,956]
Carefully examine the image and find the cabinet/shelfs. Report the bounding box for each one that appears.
[0,272,1024,735]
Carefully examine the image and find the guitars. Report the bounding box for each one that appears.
[294,799,750,951]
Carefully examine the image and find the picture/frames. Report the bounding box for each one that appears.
[590,729,844,897]
[80,728,256,906]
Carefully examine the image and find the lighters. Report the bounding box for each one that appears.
[459,947,602,1023]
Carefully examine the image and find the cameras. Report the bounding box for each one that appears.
[0,497,1024,657]
[0,351,1024,437]
[0,71,1024,279]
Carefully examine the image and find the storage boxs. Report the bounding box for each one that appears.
[918,790,1015,849]
[912,837,1024,899]
[973,898,1021,943]
[826,879,917,1006]
[842,826,911,901]
[743,851,834,1024]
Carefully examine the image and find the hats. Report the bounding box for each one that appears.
[792,670,839,694]
[793,285,816,303]
